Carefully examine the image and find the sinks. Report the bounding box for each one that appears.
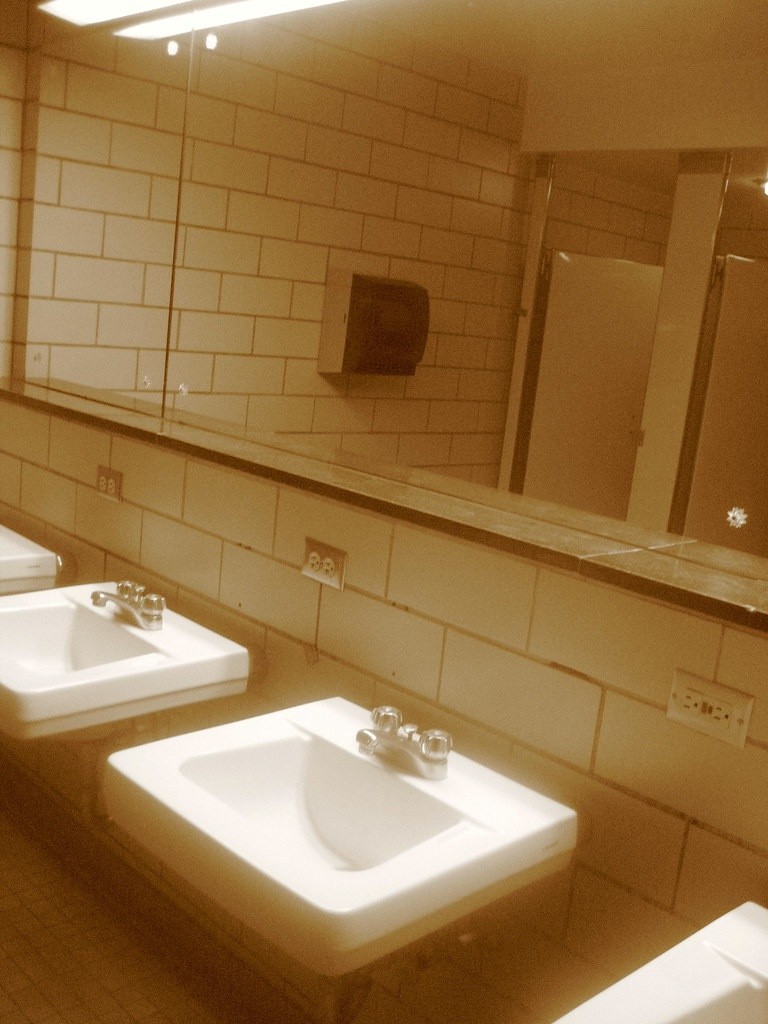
[102,714,477,978]
[0,581,172,740]
[550,900,768,1024]
[0,522,59,594]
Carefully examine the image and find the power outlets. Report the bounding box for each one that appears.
[95,465,123,502]
[665,669,753,750]
[301,536,347,590]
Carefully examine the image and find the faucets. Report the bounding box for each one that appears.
[89,580,167,630]
[353,706,453,779]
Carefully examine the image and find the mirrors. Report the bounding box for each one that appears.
[0,0,768,633]
